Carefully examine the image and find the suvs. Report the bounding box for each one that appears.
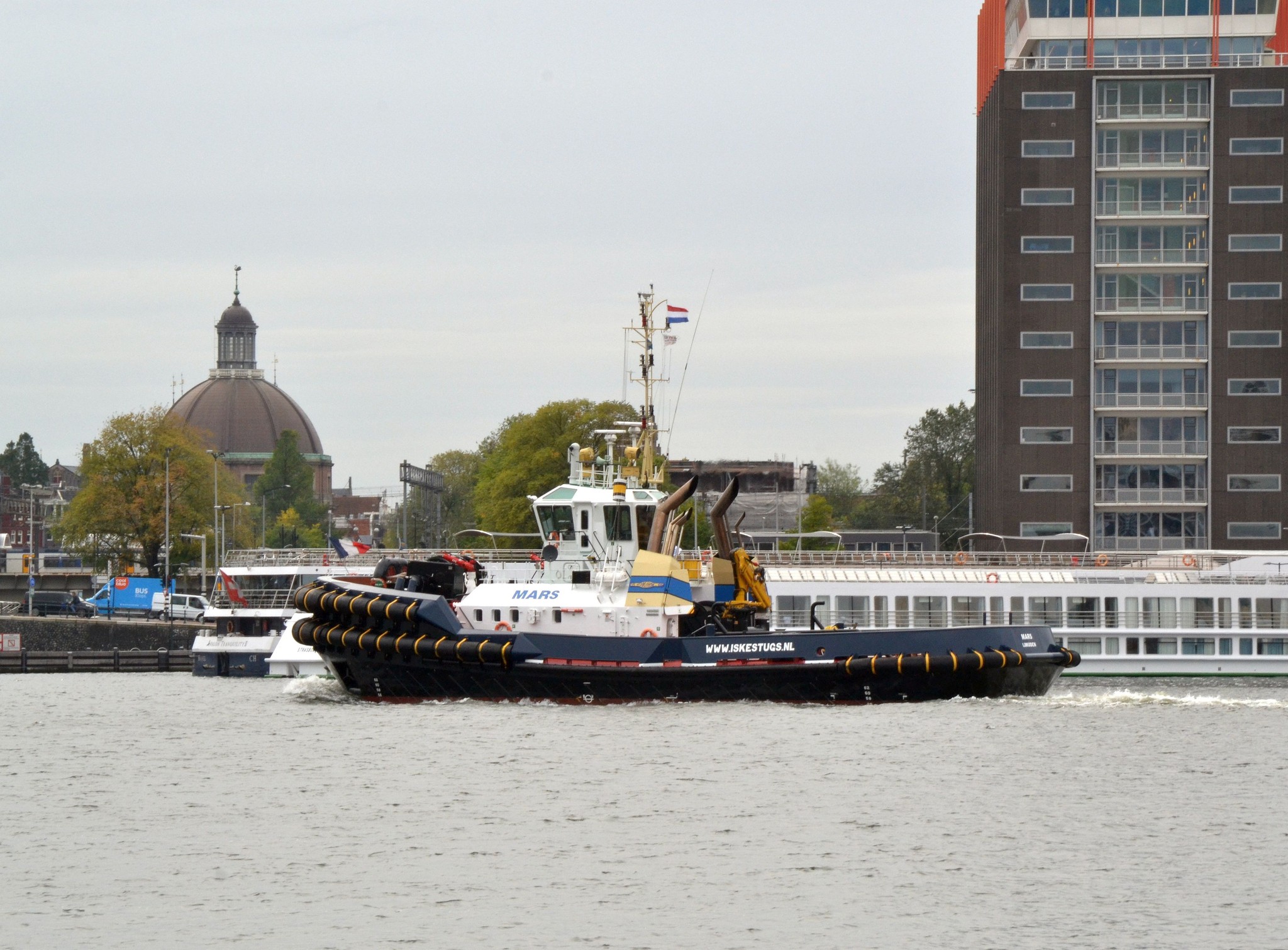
[23,591,99,618]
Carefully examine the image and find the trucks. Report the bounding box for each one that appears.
[85,575,177,619]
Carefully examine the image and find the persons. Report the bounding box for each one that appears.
[68,591,80,614]
[1027,52,1035,69]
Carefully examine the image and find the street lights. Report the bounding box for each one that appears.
[933,515,939,555]
[798,464,807,551]
[232,500,251,561]
[213,504,234,595]
[205,449,226,608]
[164,447,173,622]
[328,503,337,554]
[894,524,914,555]
[411,511,418,549]
[774,471,780,553]
[153,562,190,651]
[261,484,291,564]
[20,483,42,616]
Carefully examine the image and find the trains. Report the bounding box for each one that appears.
[0,547,166,573]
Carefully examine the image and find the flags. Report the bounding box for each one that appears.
[667,305,689,323]
[220,569,247,606]
[400,539,407,549]
[328,537,371,559]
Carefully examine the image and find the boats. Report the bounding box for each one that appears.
[291,283,1083,705]
[186,530,1288,678]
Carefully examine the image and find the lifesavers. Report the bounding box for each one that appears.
[463,550,474,559]
[495,622,512,633]
[640,629,658,638]
[370,578,387,588]
[227,621,234,633]
[548,533,560,549]
[702,549,713,564]
[1097,554,1109,566]
[444,552,476,572]
[987,572,999,584]
[956,552,967,566]
[1182,554,1193,566]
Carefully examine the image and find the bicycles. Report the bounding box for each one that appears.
[58,599,89,619]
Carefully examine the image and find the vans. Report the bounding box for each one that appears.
[152,592,216,623]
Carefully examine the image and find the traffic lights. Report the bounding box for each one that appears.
[160,574,165,588]
[167,575,171,589]
